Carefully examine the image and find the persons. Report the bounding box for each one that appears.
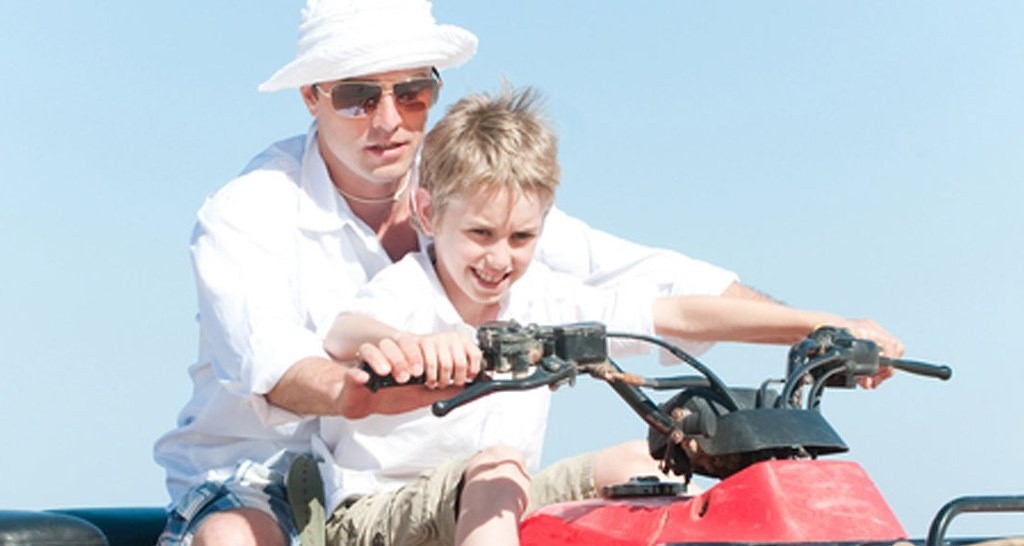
[282,74,894,546]
[151,0,904,546]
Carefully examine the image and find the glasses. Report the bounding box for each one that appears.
[315,67,443,119]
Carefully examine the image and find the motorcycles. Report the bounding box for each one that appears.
[0,325,1024,546]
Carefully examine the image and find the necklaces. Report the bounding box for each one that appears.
[333,168,411,203]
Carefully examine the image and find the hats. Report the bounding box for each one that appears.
[260,0,479,91]
